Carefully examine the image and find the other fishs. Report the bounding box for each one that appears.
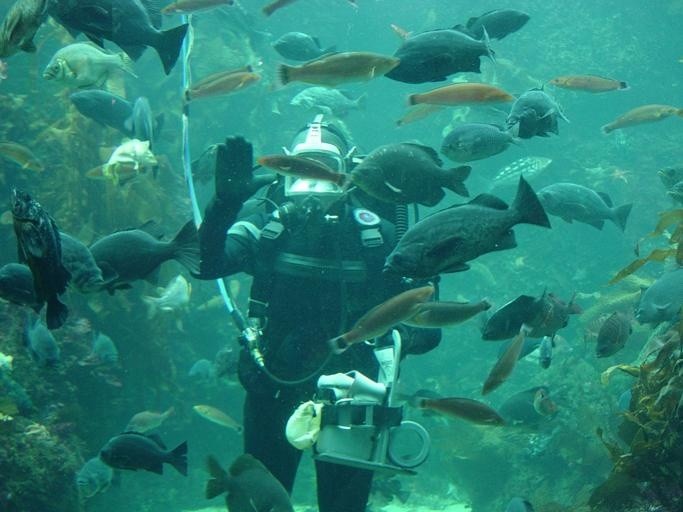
[0,0,683,512]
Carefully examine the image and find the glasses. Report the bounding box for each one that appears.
[288,148,345,180]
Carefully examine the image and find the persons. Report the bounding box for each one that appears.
[188,114,441,511]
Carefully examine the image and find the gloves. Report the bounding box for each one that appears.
[215,135,276,205]
